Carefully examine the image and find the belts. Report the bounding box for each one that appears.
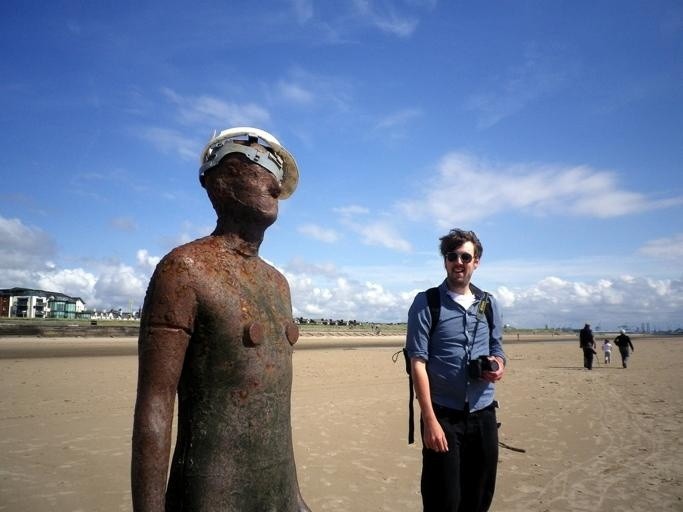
[489,400,501,408]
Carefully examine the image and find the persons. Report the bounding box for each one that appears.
[131,140,312,512]
[579,324,634,370]
[404,229,506,512]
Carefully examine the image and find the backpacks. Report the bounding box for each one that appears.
[403,286,494,406]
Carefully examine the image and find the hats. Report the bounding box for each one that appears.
[585,323,590,325]
[621,329,626,334]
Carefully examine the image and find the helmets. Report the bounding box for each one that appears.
[199,127,299,200]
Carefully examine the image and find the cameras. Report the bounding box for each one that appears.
[470,354,499,373]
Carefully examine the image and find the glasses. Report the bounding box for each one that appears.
[445,252,476,263]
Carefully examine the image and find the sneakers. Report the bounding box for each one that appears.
[588,368,593,370]
[623,363,627,368]
[605,360,607,363]
